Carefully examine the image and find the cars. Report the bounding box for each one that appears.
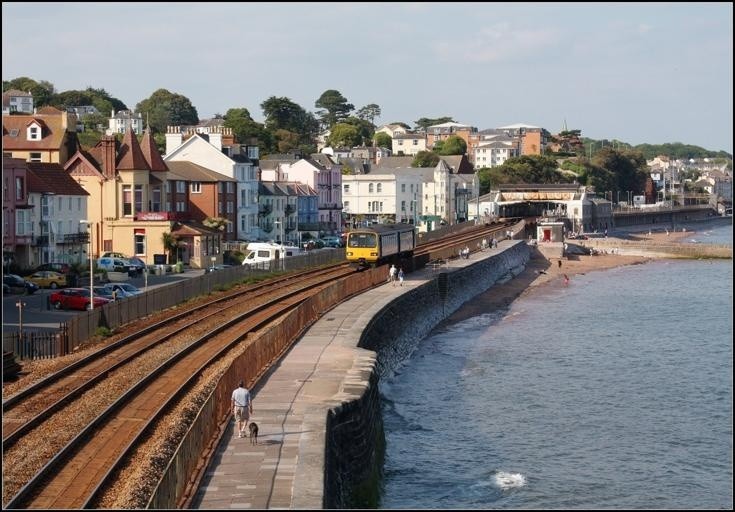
[302,239,324,249]
[50,282,143,312]
[4,274,38,295]
[206,264,232,274]
[24,271,66,289]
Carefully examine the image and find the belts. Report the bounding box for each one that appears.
[235,405,248,407]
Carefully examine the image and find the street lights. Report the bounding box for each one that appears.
[274,221,283,270]
[80,219,94,308]
[136,233,148,287]
[605,190,646,210]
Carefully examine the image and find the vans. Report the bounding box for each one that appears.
[322,237,344,248]
[97,251,146,276]
[242,246,300,273]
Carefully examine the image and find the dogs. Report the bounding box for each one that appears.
[249,423,259,446]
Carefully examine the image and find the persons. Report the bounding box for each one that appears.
[605,228,608,238]
[489,237,493,248]
[389,264,397,286]
[398,268,405,286]
[465,246,469,258]
[563,274,569,284]
[482,238,487,248]
[511,230,514,239]
[506,230,510,240]
[494,239,497,248]
[230,380,252,438]
[460,248,464,260]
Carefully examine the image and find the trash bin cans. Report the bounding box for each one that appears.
[176,262,183,273]
[153,264,165,276]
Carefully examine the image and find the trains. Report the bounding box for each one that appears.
[345,223,417,268]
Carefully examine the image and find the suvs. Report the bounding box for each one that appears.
[29,263,80,287]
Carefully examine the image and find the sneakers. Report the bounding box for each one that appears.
[239,431,247,438]
